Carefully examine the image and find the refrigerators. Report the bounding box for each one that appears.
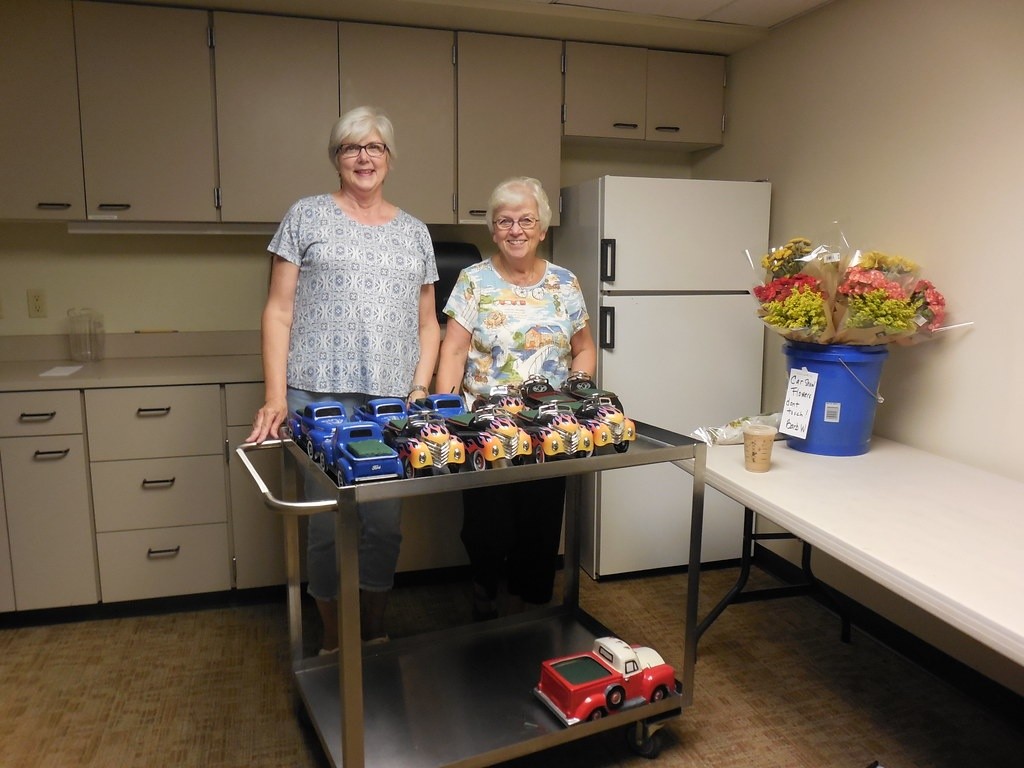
[552,176,774,581]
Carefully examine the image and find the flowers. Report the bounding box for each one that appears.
[754,239,973,346]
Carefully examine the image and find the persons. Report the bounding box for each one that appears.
[245,107,442,660]
[435,175,596,624]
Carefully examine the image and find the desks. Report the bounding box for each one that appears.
[669,433,1024,696]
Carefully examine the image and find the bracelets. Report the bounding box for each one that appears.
[408,385,429,399]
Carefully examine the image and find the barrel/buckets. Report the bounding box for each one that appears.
[780,337,889,457]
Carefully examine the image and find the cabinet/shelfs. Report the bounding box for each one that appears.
[0,390,98,614]
[213,10,456,223]
[0,0,218,224]
[85,384,233,604]
[225,385,566,591]
[457,31,565,226]
[234,418,707,768]
[562,41,727,154]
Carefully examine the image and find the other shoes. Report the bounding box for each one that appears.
[361,633,390,648]
[471,601,499,624]
[318,647,340,654]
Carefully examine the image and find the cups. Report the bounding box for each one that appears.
[743,423,777,472]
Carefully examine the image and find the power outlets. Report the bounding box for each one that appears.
[27,286,47,318]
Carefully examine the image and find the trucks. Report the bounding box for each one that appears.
[287,400,349,464]
[470,393,532,413]
[518,380,577,413]
[445,408,533,473]
[408,393,469,418]
[513,403,594,465]
[533,636,675,730]
[572,398,638,453]
[320,420,404,487]
[348,398,414,439]
[558,370,625,418]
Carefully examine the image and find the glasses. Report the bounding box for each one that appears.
[494,217,540,229]
[336,142,389,157]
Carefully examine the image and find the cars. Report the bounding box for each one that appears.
[381,410,465,479]
